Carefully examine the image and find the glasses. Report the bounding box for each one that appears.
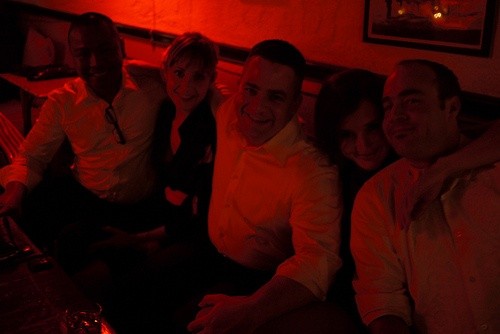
[104,105,127,144]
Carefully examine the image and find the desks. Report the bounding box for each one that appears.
[0,215,90,334]
[0,72,81,137]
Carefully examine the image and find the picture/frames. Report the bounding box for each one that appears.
[363,0,497,58]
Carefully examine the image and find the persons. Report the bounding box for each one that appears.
[89,31,218,334]
[123,39,342,334]
[0,11,167,301]
[349,59,500,334]
[313,67,500,334]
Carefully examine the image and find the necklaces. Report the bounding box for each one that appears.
[171,125,180,134]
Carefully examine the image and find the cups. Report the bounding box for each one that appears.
[67,301,103,334]
